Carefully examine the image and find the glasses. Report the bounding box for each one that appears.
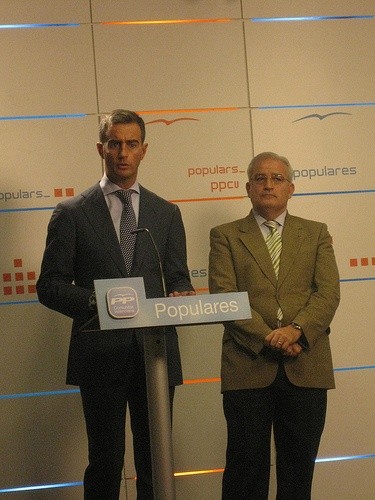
[250,172,291,186]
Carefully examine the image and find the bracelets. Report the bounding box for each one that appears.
[291,323,303,330]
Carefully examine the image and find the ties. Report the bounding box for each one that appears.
[264,221,283,284]
[114,189,137,278]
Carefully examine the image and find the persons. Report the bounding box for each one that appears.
[37,109,198,500]
[208,152,342,500]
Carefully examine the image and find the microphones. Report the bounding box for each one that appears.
[131,227,167,297]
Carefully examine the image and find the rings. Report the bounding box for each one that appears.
[277,341,283,346]
